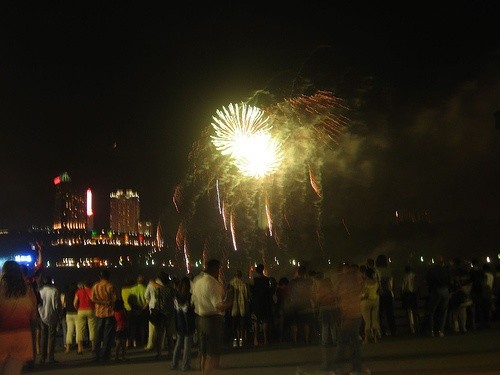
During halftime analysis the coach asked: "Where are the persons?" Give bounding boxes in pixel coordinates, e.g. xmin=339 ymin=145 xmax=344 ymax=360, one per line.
xmin=19 ymin=242 xmax=500 ymax=374
xmin=0 ymin=259 xmax=42 ymax=374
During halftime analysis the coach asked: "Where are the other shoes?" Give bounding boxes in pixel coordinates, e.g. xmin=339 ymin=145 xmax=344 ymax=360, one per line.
xmin=252 ymin=340 xmax=258 ymax=346
xmin=233 ymin=340 xmax=238 ymax=347
xmin=167 ymin=353 xmax=172 ymax=360
xmin=239 ymin=339 xmax=245 ymax=347
xmin=38 ymin=360 xmax=46 ymax=367
xmin=76 ymin=349 xmax=84 ymax=356
xmin=169 ymin=364 xmax=179 ymax=371
xmin=263 ymin=339 xmax=268 ymax=346
xmin=181 ymin=364 xmax=192 ymax=371
xmin=47 ymin=360 xmax=56 ymax=367
xmin=155 ymin=354 xmax=161 ymax=360
xmin=64 ymin=350 xmax=72 ymax=354
